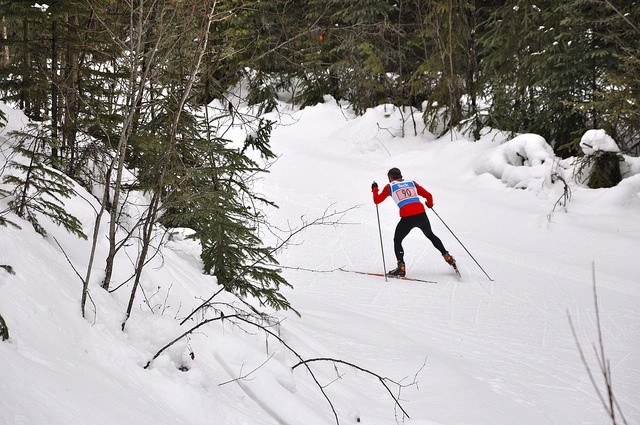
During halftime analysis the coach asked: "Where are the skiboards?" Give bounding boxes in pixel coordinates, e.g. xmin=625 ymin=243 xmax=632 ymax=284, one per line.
xmin=365 ymin=264 xmax=461 ymax=283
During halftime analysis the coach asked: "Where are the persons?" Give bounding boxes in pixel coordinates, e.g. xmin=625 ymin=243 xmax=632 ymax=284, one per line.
xmin=371 ymin=167 xmax=457 ymax=276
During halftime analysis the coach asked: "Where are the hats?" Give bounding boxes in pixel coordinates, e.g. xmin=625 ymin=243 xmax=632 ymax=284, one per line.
xmin=388 ymin=168 xmax=402 ymax=177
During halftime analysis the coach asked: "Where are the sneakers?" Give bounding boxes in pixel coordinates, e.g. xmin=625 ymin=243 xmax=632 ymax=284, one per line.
xmin=389 ymin=262 xmax=405 ymax=277
xmin=443 ymin=252 xmax=456 ymax=266
